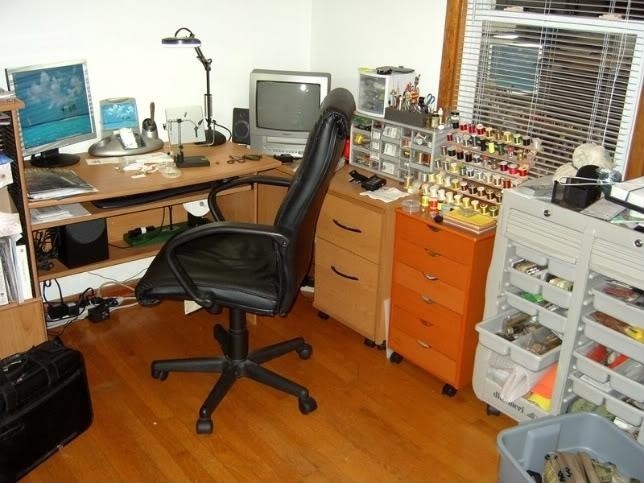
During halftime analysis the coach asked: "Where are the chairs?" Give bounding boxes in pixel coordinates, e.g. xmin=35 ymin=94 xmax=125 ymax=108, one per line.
xmin=136 ymin=87 xmax=352 ymax=432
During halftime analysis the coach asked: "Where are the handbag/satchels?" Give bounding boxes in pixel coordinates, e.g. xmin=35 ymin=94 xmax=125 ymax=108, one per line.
xmin=0 ymin=336 xmax=94 ymax=482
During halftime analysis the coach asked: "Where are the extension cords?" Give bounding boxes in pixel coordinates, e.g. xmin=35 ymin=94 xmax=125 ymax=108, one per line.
xmin=46 ymin=297 xmax=121 ymax=328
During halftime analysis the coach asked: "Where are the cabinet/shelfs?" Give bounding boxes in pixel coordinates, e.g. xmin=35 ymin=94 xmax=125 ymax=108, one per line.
xmin=387 ymin=197 xmax=496 ymax=395
xmin=473 ymin=166 xmax=644 ymax=446
xmin=0 ymin=94 xmax=48 ymax=357
xmin=419 ymin=116 xmax=543 ymax=218
xmin=255 ymin=155 xmax=315 ymax=284
xmin=348 ymin=102 xmax=452 ymax=187
xmin=315 ymin=161 xmax=421 ymax=350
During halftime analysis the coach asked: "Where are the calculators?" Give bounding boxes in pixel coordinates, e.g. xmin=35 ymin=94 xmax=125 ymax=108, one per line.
xmin=362 ymin=174 xmax=387 ymax=191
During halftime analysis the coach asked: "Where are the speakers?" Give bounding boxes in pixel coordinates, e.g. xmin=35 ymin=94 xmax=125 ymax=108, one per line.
xmin=53 ymin=218 xmax=109 ymax=269
xmin=232 ymin=107 xmax=250 ymax=144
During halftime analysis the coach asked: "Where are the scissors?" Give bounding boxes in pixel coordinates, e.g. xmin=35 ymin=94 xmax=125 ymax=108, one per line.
xmin=426 ymin=93 xmax=435 ymax=105
xmin=228 ymin=155 xmax=246 ymax=164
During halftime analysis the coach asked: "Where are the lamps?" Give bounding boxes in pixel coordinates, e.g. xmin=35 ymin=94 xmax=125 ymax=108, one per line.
xmin=159 ymin=27 xmax=234 ymax=146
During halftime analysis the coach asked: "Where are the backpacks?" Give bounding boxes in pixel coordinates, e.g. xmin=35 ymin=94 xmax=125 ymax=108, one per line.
xmin=526 ymin=450 xmax=626 ymax=482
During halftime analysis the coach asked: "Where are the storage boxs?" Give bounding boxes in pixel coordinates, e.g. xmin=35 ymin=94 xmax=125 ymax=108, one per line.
xmin=385 ymin=106 xmax=434 ymax=127
xmin=493 ymin=411 xmax=642 ymax=482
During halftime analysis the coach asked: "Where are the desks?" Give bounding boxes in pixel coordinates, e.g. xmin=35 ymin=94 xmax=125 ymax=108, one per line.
xmin=20 ymin=134 xmax=283 ymax=325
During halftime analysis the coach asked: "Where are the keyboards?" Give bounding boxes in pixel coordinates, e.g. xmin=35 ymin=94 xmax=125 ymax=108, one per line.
xmin=92 ymin=182 xmax=209 ymax=209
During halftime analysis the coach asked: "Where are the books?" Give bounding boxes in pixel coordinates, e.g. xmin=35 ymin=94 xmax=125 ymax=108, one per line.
xmin=23 ymin=168 xmax=97 ymax=198
xmin=0 ymin=238 xmax=29 ymax=306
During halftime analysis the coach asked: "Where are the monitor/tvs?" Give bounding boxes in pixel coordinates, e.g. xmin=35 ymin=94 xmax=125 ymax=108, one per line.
xmin=6 ymin=58 xmax=97 ymax=168
xmin=248 ymin=68 xmax=331 ymax=158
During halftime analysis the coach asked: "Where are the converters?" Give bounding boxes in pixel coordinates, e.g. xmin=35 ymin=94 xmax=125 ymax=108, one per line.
xmin=88 ymin=305 xmax=109 ymax=323
xmin=65 ymin=301 xmax=79 ymax=315
xmin=50 ymin=302 xmax=65 ymax=318
